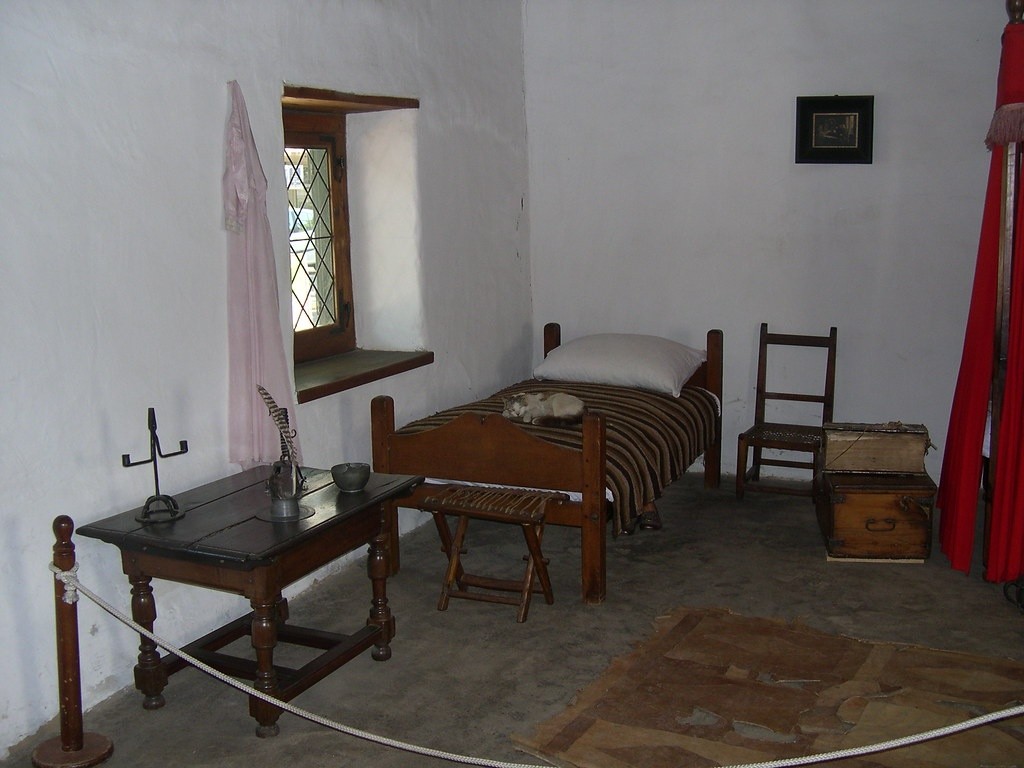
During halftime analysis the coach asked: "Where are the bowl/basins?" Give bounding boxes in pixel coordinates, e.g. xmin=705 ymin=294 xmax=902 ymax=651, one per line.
xmin=331 ymin=463 xmax=371 ymax=492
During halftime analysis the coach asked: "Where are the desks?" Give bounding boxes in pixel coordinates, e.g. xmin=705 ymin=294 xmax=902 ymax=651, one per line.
xmin=74 ymin=463 xmax=425 ymax=737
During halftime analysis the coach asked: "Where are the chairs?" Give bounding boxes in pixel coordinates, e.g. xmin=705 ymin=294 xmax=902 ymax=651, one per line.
xmin=733 ymin=322 xmax=838 ymax=501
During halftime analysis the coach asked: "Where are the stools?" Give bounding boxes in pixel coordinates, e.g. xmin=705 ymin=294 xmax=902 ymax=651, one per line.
xmin=421 ymin=484 xmax=570 ymax=622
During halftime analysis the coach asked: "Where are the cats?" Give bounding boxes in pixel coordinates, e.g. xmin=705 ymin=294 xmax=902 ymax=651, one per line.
xmin=500 ymin=390 xmax=587 ymax=428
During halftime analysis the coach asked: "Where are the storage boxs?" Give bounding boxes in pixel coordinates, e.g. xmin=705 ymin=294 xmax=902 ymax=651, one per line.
xmin=815 ymin=422 xmax=938 ymax=561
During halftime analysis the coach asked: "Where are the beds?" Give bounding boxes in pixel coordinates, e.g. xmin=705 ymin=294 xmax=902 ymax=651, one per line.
xmin=369 ymin=321 xmax=724 ymax=602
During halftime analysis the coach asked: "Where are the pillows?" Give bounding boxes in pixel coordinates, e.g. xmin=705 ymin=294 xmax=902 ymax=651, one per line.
xmin=533 ymin=333 xmax=708 ymax=398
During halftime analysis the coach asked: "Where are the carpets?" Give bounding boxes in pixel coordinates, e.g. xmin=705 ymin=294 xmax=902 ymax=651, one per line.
xmin=510 ymin=605 xmax=1024 ymax=768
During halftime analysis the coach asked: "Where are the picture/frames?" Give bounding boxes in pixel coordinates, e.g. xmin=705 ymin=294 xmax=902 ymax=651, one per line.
xmin=795 ymin=94 xmax=874 ymax=164
